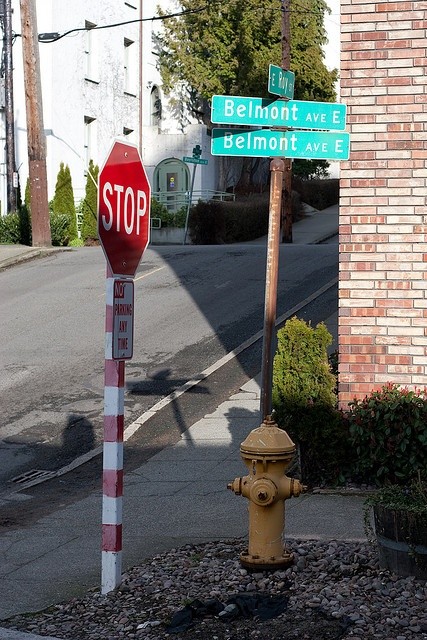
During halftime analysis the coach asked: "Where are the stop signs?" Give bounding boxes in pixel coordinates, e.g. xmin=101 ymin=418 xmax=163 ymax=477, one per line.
xmin=97 ymin=141 xmax=152 ymax=279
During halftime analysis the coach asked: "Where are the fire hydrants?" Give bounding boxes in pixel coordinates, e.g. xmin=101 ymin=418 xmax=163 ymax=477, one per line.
xmin=228 ymin=410 xmax=311 ymax=569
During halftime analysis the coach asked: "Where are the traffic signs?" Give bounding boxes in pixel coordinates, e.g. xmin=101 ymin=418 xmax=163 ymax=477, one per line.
xmin=201 ymin=126 xmax=350 ymax=162
xmin=208 ymin=92 xmax=349 ymax=129
xmin=269 ymin=63 xmax=297 ymax=99
xmin=183 ymin=156 xmax=207 ymax=165
